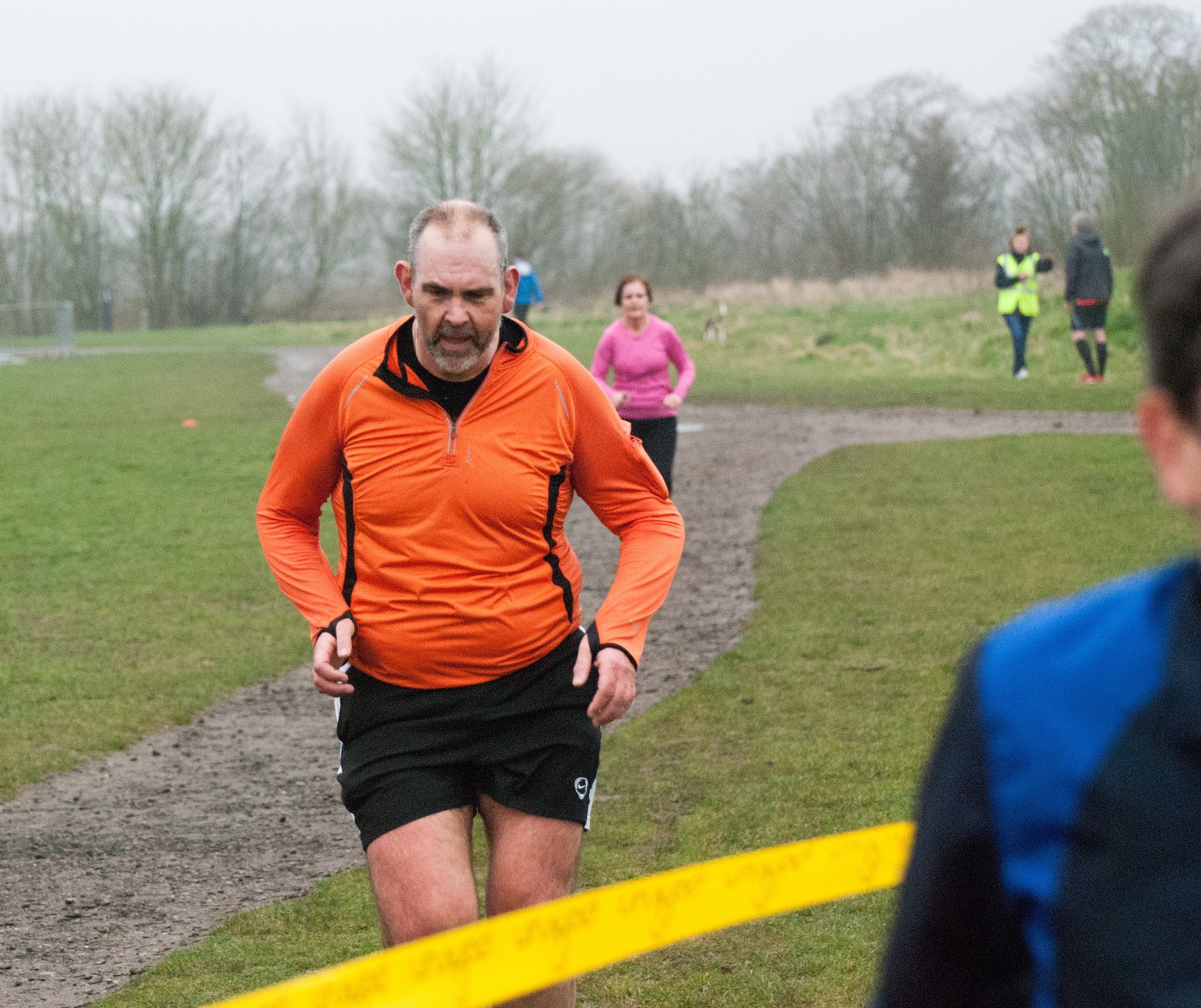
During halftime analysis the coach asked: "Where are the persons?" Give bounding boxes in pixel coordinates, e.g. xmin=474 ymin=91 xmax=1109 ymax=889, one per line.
xmin=878 ymin=203 xmax=1201 ymax=1008
xmin=994 ymin=226 xmax=1054 ymax=380
xmin=1063 ymin=211 xmax=1114 ymax=380
xmin=253 ymin=200 xmax=695 ymax=1008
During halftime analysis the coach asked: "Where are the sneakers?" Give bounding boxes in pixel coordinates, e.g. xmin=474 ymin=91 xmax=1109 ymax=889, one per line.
xmin=1014 ymin=368 xmax=1028 ymax=379
xmin=1080 ymin=372 xmax=1096 ymax=384
xmin=1093 ymin=374 xmax=1104 ymax=381
